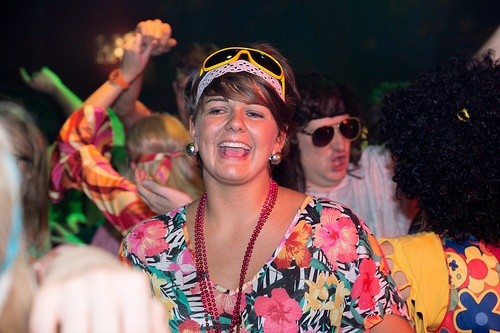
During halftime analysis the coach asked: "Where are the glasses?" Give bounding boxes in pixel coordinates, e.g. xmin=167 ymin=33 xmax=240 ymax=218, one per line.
xmin=200 ymin=46 xmax=285 ymax=102
xmin=300 ymin=117 xmax=362 ymax=148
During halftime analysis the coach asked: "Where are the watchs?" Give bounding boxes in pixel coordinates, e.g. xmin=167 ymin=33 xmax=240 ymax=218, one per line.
xmin=109 ymin=69 xmax=128 ymax=89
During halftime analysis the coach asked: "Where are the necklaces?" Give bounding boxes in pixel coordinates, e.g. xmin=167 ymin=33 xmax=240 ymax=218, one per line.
xmin=193 ymin=180 xmax=280 ymax=333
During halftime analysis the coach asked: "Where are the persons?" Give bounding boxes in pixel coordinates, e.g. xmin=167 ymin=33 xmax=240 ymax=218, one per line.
xmin=0 ymin=101 xmax=173 ymax=333
xmin=19 ymin=18 xmax=413 ymax=258
xmin=112 ymin=43 xmax=412 ymax=333
xmin=374 ymin=27 xmax=500 ymax=333
